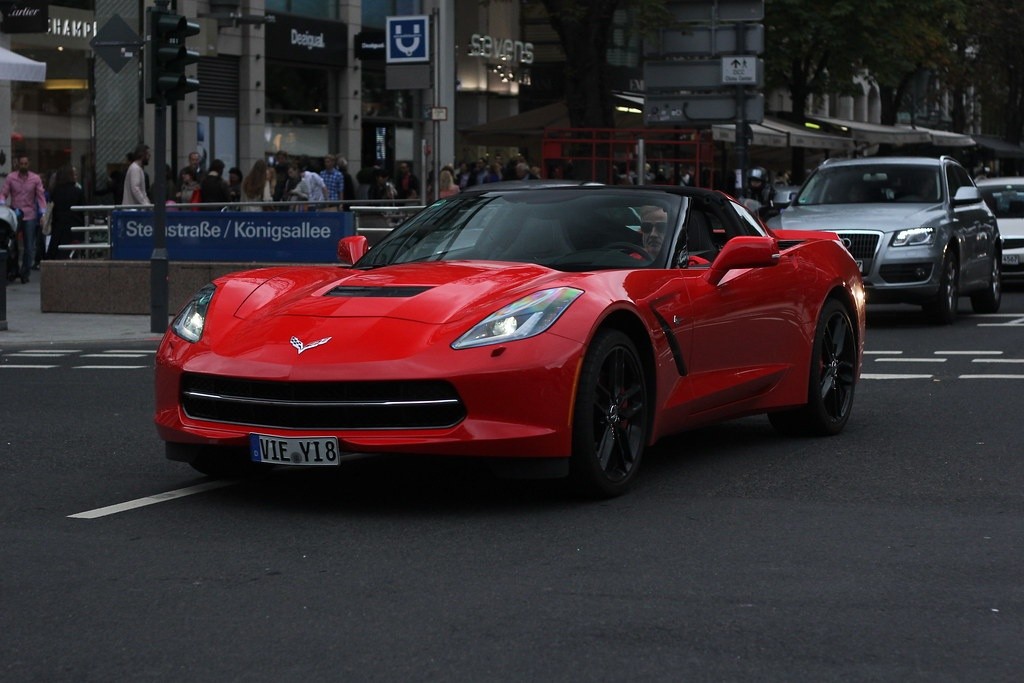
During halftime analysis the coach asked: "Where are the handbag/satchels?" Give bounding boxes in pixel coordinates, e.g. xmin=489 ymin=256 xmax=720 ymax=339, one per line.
xmin=191 ymin=187 xmax=203 ymax=211
xmin=41 ymin=202 xmax=54 ymax=235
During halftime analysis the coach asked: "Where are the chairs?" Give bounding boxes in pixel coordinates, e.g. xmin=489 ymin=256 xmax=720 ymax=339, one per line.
xmin=482 ymin=215 xmax=721 ymax=272
xmin=983 ymin=195 xmax=1024 ymax=219
xmin=837 ymin=174 xmax=957 ymax=205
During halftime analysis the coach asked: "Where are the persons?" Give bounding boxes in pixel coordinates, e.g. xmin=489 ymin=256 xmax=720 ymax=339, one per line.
xmin=48 ymin=145 xmax=460 ymax=260
xmin=629 ymin=204 xmax=712 ymax=266
xmin=0 ymin=154 xmax=47 ymax=285
xmin=897 ymin=176 xmax=939 ymax=201
xmin=455 ymin=152 xmax=808 ymax=208
xmin=832 ymin=183 xmax=867 ymax=205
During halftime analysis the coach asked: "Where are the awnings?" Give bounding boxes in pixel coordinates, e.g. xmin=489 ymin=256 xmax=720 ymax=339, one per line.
xmin=710 ymin=116 xmax=1024 ymax=159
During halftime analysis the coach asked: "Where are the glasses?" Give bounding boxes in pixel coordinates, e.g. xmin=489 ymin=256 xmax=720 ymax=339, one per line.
xmin=641 ymin=222 xmax=667 ymax=234
xmin=399 ymin=166 xmax=406 ymax=170
xmin=19 ymin=162 xmax=28 ymax=166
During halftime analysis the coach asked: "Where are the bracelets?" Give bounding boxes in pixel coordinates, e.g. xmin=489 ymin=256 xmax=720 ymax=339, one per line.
xmin=41 ymin=214 xmax=46 ymax=217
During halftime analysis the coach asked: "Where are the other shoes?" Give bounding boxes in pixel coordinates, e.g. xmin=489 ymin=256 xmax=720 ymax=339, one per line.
xmin=21 ymin=276 xmax=30 ymax=284
xmin=32 ymin=264 xmax=39 ymax=271
xmin=8 ymin=273 xmax=16 ymax=282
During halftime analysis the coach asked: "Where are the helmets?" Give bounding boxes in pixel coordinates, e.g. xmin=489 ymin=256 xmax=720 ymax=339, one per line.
xmin=746 ymin=166 xmax=768 ymax=183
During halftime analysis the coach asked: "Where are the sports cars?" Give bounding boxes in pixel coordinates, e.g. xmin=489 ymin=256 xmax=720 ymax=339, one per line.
xmin=156 ymin=186 xmax=863 ymax=503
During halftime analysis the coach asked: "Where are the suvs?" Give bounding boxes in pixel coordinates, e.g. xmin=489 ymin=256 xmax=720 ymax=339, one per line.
xmin=975 ymin=174 xmax=1023 ymax=290
xmin=764 ymin=156 xmax=1002 ymax=317
